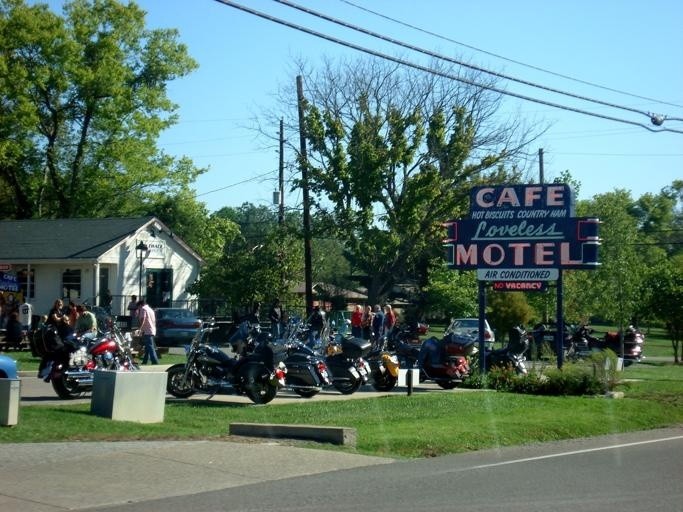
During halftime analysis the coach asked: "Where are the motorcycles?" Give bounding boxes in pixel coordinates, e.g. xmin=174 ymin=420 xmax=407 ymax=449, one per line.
xmin=0 ymin=356 xmax=17 ymax=378
xmin=567 ymin=323 xmax=645 ymax=367
xmin=32 ymin=315 xmax=139 ymax=399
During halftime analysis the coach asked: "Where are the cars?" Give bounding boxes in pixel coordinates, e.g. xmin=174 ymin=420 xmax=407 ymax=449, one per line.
xmin=152 ymin=307 xmax=202 ymax=346
xmin=528 ymin=321 xmax=575 ymax=360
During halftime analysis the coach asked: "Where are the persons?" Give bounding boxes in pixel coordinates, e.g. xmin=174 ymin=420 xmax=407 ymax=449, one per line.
xmin=249 ymin=297 xmax=396 ymax=352
xmin=6 ymin=288 xmax=159 ymax=364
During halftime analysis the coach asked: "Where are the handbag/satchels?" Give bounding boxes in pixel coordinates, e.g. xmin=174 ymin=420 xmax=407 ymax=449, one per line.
xmin=28 ymin=326 xmax=64 ymax=357
